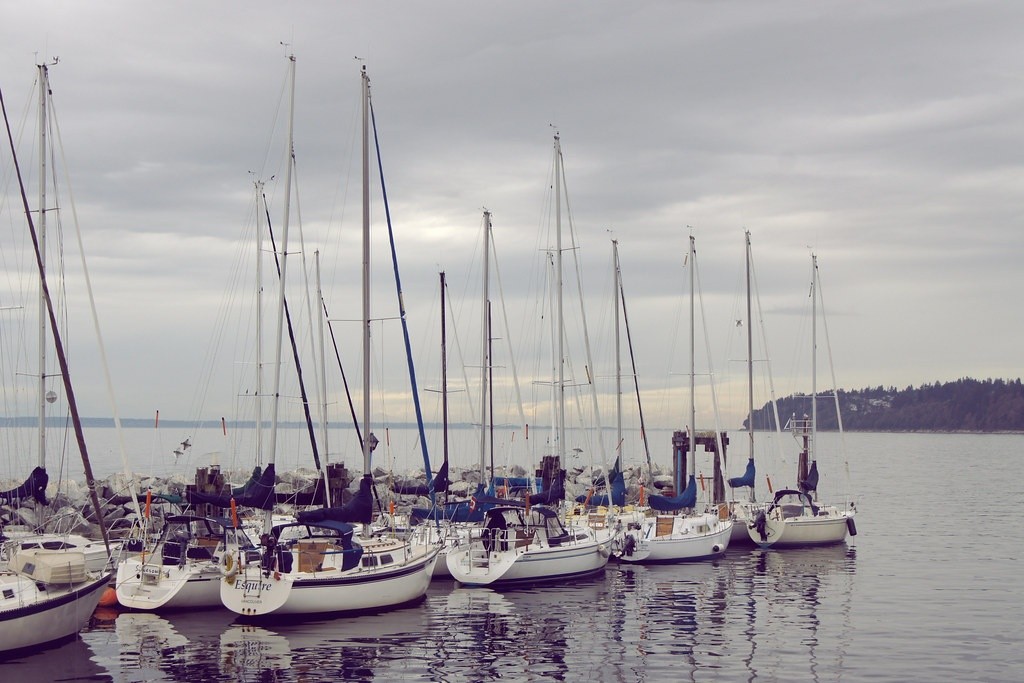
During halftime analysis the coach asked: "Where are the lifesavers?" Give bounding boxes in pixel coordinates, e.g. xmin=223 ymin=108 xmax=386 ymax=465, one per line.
xmin=377 ymin=513 xmax=391 ymax=527
xmin=219 ymin=551 xmax=238 ymax=576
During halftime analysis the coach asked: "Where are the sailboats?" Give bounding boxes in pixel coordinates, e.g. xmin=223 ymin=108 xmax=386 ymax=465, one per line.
xmin=0 ymin=44 xmax=858 ymax=663
